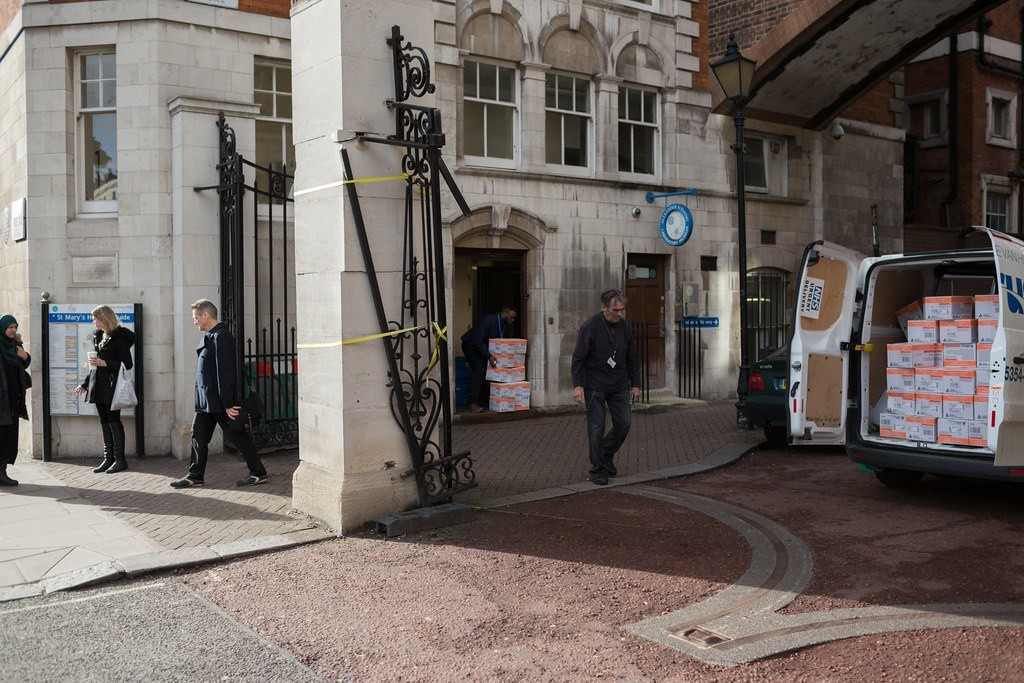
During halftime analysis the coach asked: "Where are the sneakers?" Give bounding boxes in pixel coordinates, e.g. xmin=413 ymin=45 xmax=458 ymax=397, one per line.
xmin=238 ymin=474 xmax=269 ymax=486
xmin=171 ymin=474 xmax=204 ymax=488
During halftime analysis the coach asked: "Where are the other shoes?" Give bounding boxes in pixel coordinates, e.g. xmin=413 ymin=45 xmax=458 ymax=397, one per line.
xmin=592 ymin=475 xmax=609 ymax=485
xmin=604 ymin=454 xmax=617 ymax=477
xmin=465 ymin=404 xmax=484 ymax=413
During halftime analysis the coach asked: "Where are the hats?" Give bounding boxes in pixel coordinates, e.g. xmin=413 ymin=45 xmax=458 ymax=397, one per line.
xmin=0 ymin=313 xmax=19 ymax=337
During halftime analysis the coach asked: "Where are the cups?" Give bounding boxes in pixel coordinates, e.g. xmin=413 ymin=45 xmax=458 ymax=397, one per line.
xmin=88 ymin=351 xmax=97 ymax=370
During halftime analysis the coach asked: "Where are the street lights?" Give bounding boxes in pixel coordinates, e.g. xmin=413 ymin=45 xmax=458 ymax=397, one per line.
xmin=707 ymin=28 xmax=760 ymax=430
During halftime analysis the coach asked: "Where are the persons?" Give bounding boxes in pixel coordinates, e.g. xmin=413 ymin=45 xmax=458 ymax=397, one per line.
xmin=571 ymin=290 xmax=641 ymax=485
xmin=75 ymin=304 xmax=137 ymax=473
xmin=0 ymin=314 xmax=32 ymax=486
xmin=170 ymin=298 xmax=270 ymax=489
xmin=460 ymin=307 xmax=516 ymax=412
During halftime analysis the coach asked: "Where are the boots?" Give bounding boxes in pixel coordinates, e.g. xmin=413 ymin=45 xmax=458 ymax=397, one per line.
xmin=105 ymin=422 xmax=128 ymax=473
xmin=93 ymin=423 xmax=115 ymax=473
xmin=0 ymin=463 xmax=19 ymax=486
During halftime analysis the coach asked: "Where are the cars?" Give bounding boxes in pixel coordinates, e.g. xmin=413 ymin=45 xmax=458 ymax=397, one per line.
xmin=745 ymin=339 xmax=788 ymax=446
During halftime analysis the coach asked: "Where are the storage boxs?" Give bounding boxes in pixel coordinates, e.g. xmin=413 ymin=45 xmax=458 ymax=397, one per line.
xmin=490 ymin=396 xmax=529 ymax=411
xmin=490 ymin=382 xmax=531 ymax=397
xmin=489 ymin=338 xmax=527 ymax=354
xmin=455 ymin=356 xmax=472 ymax=406
xmin=486 ymin=367 xmax=526 ymax=382
xmin=879 ymin=293 xmax=1000 ymax=446
xmin=487 ymin=354 xmax=526 ymax=368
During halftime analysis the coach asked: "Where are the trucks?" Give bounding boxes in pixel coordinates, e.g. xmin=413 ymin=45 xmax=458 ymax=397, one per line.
xmin=781 ymin=225 xmax=1024 ymax=490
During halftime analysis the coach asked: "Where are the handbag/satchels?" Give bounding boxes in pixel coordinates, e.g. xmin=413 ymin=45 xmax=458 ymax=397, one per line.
xmin=233 ymin=392 xmax=263 ymax=431
xmin=110 ymin=363 xmax=138 ymax=410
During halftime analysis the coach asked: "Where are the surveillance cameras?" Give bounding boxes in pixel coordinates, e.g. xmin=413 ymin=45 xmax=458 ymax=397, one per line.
xmin=831 ymin=125 xmax=844 ymax=140
xmin=632 ymin=207 xmax=642 ymax=217
